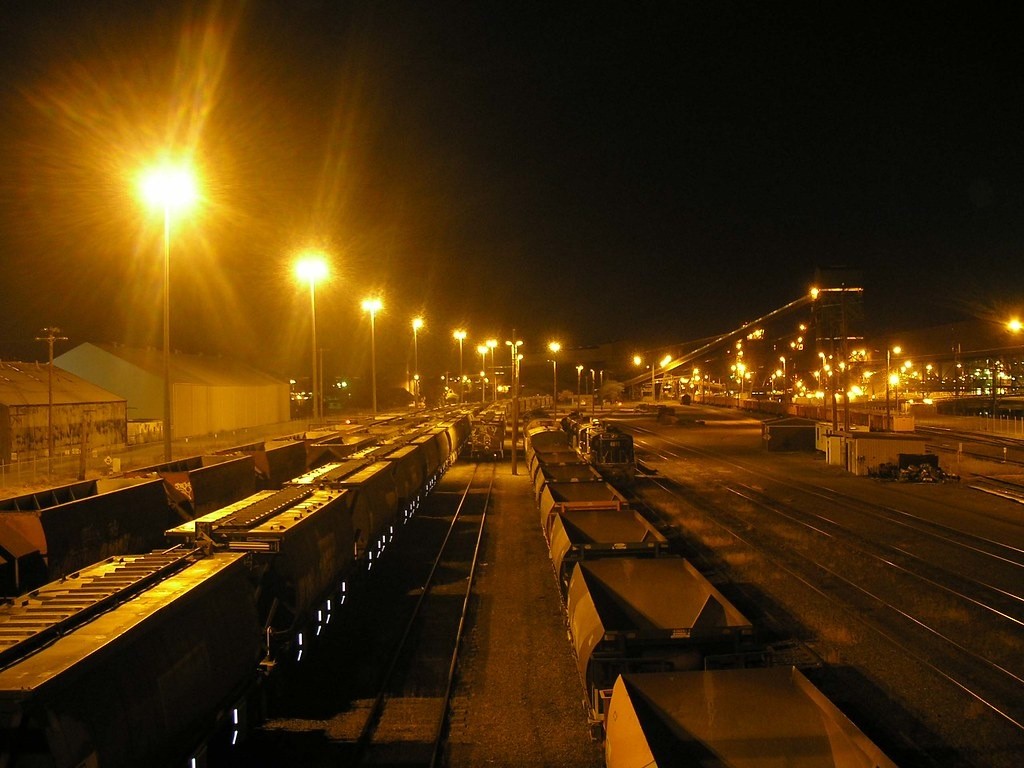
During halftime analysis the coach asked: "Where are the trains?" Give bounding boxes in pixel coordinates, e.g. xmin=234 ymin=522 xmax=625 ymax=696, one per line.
xmin=693 ymin=392 xmax=916 ymax=433
xmin=1 ymin=399 xmax=491 ymax=768
xmin=467 ymin=391 xmax=552 ymax=462
xmin=522 ymin=406 xmax=898 ymax=768
xmin=1 ymin=401 xmax=476 ymax=605
xmin=561 ymin=410 xmax=636 ymax=490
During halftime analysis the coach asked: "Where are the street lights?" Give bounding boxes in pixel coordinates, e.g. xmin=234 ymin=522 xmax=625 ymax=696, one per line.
xmin=779 ymin=356 xmax=786 ymax=393
xmin=412 ymin=316 xmax=426 ymax=408
xmin=599 ymin=369 xmax=605 ymax=410
xmin=34 ymin=325 xmax=69 ymax=486
xmin=544 ymin=359 xmax=558 ymax=422
xmin=886 ymin=346 xmax=901 ymax=433
xmin=819 ymin=351 xmax=825 ymax=386
xmin=293 ymin=250 xmax=330 ymax=419
xmin=504 ymin=340 xmax=525 ymax=397
xmin=889 ymin=373 xmax=899 ymax=411
xmin=359 ymin=297 xmax=385 ymax=414
xmin=548 ymin=342 xmax=560 ymax=395
xmin=135 ymin=158 xmax=201 ymax=463
xmin=589 ymin=368 xmax=595 ymax=415
xmin=771 ymin=373 xmax=776 ymax=400
xmin=486 ymin=338 xmax=498 ymax=400
xmin=575 ymin=365 xmax=583 ymax=408
xmin=817 ymin=364 xmax=830 ymax=388
xmin=477 ymin=345 xmax=488 ymax=403
xmin=453 ymin=329 xmax=468 ymax=403
